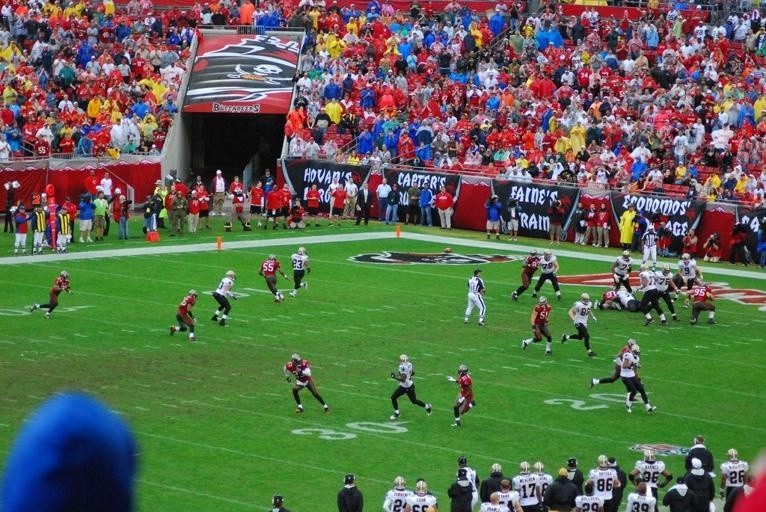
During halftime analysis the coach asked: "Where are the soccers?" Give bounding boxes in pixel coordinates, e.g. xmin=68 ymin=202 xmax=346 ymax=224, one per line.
xmin=445 ymin=248 xmax=451 ymax=252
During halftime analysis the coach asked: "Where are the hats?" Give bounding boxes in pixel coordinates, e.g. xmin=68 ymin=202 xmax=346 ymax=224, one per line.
xmin=0 ymin=0 xmax=766 ymax=247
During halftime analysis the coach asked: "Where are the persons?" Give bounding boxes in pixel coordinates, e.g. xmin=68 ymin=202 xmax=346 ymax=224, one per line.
xmin=446 ymin=365 xmax=475 ymax=427
xmin=211 ymin=270 xmax=238 ymax=328
xmin=270 ymin=436 xmax=766 ymax=511
xmin=1 ymin=2 xmax=766 ymax=270
xmin=30 ymin=271 xmax=72 ymax=319
xmin=1 ymin=391 xmax=140 ymax=511
xmin=511 ymin=250 xmax=718 ymax=356
xmin=258 ymin=247 xmax=311 ymax=303
xmin=464 ymin=269 xmax=488 ymax=326
xmin=283 ymin=354 xmax=329 ymax=413
xmin=589 ymin=339 xmax=657 ymax=415
xmin=170 ymin=289 xmax=198 ymax=341
xmin=390 ymin=354 xmax=433 ymax=420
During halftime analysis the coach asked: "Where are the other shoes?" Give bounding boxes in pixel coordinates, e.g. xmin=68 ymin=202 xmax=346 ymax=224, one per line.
xmin=29 ymin=248 xmax=720 ymax=430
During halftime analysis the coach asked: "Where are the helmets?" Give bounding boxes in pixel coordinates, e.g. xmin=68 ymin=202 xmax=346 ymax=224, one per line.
xmin=269 ymin=434 xmax=740 ymax=512
